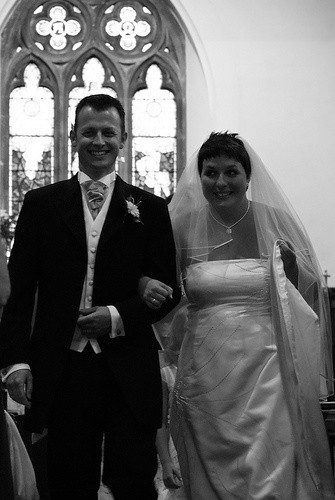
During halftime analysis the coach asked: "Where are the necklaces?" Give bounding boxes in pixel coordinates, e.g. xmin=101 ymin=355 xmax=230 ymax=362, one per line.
xmin=210 ymin=200 xmax=250 ymax=235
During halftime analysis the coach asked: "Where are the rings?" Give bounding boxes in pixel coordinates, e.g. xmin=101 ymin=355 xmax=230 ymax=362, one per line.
xmin=152 ymin=299 xmax=155 ymax=304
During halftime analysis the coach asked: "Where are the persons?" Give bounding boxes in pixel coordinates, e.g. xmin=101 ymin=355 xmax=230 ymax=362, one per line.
xmin=137 ymin=130 xmax=334 ymax=500
xmin=0 ymin=237 xmax=39 ymax=500
xmin=0 ymin=94 xmax=182 ymax=500
xmin=153 ymin=303 xmax=192 ymax=500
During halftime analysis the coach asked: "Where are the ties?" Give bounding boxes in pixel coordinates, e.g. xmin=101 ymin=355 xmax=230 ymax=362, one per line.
xmin=81 ymin=180 xmax=108 ymax=220
